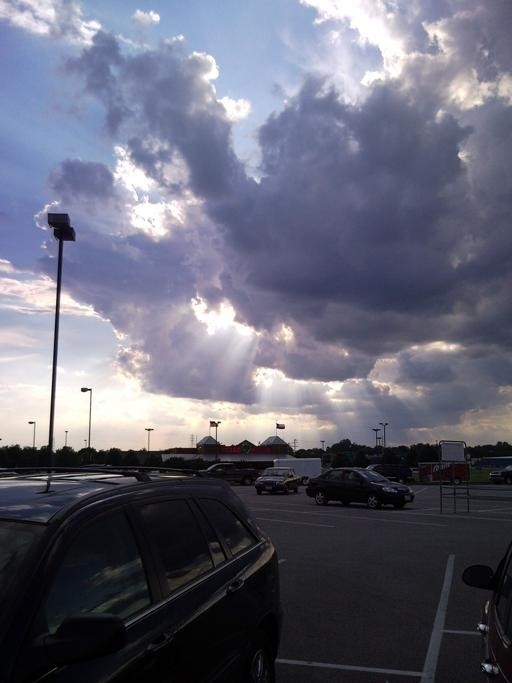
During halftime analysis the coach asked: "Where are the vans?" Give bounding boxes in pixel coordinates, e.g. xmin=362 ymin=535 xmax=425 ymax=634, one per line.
xmin=365 ymin=462 xmax=414 ymax=482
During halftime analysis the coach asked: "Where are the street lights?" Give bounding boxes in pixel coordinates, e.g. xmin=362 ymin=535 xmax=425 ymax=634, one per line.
xmin=80 ymin=387 xmax=92 ymax=447
xmin=65 ymin=429 xmax=69 ymax=444
xmin=378 ymin=421 xmax=389 ymax=446
xmin=377 ymin=437 xmax=382 ymax=446
xmin=371 ymin=427 xmax=382 ymax=446
xmin=144 ymin=427 xmax=154 ymax=450
xmin=28 ymin=420 xmax=37 ymax=447
xmin=46 ymin=211 xmax=80 ymax=465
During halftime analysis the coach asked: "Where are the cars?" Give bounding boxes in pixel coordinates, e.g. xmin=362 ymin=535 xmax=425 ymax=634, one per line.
xmin=303 ymin=464 xmax=417 ymax=510
xmin=462 ymin=537 xmax=512 ymax=682
xmin=253 ymin=465 xmax=301 ymax=495
xmin=487 ymin=464 xmax=512 ymax=483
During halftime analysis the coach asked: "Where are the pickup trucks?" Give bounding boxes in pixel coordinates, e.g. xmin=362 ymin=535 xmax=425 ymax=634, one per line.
xmin=192 ymin=461 xmax=257 ymax=485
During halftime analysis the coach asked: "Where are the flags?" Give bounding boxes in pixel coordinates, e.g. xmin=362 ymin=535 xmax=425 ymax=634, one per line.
xmin=276 ymin=423 xmax=285 ymax=429
xmin=210 ymin=421 xmax=218 ymax=427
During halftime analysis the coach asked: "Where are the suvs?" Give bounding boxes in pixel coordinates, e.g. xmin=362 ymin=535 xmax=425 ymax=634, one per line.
xmin=0 ymin=464 xmax=288 ymax=682
